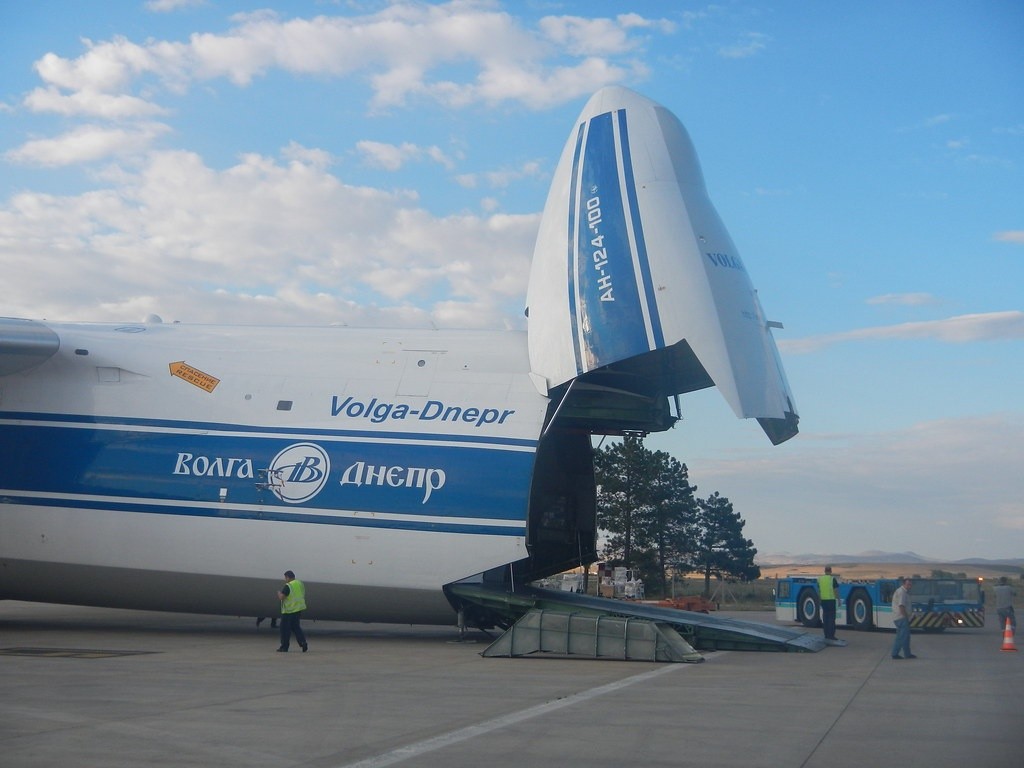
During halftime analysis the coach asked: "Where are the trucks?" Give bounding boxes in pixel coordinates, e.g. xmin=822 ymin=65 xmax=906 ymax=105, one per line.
xmin=775 ymin=572 xmax=986 ymax=631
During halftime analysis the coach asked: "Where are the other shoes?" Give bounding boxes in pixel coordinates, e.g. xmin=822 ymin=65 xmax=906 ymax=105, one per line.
xmin=271 ymin=624 xmax=279 ymax=628
xmin=906 ymin=654 xmax=916 ymax=658
xmin=302 ymin=642 xmax=307 ymax=652
xmin=892 ymin=655 xmax=904 ymax=659
xmin=825 ymin=636 xmax=838 ymax=640
xmin=277 ymin=646 xmax=287 ymax=652
xmin=256 ymin=617 xmax=260 ymax=627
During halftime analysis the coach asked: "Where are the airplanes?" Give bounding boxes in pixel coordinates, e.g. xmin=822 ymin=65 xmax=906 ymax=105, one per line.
xmin=1 ymin=81 xmax=799 ymax=642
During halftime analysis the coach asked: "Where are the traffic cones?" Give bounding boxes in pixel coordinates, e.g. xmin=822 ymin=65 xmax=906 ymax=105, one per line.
xmin=1000 ymin=616 xmax=1018 ymax=651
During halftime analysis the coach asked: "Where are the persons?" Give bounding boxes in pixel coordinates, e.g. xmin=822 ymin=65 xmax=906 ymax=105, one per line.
xmin=993 ymin=577 xmax=1017 ymax=636
xmin=892 ymin=579 xmax=916 ymax=659
xmin=276 ymin=571 xmax=308 ymax=652
xmin=819 ymin=567 xmax=841 ymax=640
